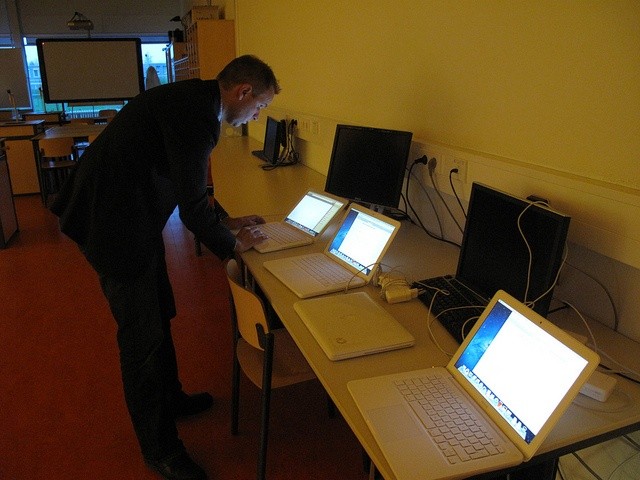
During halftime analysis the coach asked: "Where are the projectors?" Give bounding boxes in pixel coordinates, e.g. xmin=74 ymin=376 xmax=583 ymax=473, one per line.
xmin=65 ymin=20 xmax=94 ymax=30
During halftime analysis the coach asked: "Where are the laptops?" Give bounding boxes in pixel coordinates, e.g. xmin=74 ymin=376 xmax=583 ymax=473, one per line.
xmin=241 ymin=187 xmax=350 ymax=254
xmin=262 ymin=202 xmax=401 ymax=300
xmin=292 ymin=290 xmax=417 ymax=362
xmin=345 ymin=289 xmax=601 ymax=479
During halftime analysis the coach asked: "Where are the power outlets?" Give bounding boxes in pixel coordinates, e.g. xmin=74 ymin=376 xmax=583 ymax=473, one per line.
xmin=412 ymin=148 xmax=442 ymax=177
xmin=301 ymin=119 xmax=319 ymax=135
xmin=288 ymin=117 xmax=299 ymax=131
xmin=448 ymin=157 xmax=468 ymax=183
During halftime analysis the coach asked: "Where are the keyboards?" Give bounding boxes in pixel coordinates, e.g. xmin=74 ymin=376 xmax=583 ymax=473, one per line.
xmin=408 ymin=274 xmax=486 ymax=346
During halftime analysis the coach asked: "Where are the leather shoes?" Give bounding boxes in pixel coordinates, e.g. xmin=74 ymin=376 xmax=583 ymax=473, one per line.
xmin=150 ymin=439 xmax=207 ymax=480
xmin=180 ymin=393 xmax=212 ymax=416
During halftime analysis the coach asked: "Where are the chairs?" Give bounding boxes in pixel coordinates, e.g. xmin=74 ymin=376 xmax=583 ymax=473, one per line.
xmin=98 ymin=110 xmax=116 ymax=122
xmin=34 ymin=136 xmax=80 ymax=210
xmin=71 ymin=117 xmax=91 ymax=127
xmin=224 ymin=273 xmax=317 ymax=479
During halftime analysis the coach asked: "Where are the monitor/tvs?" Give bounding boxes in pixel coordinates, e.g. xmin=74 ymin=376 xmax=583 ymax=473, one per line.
xmin=454 ymin=181 xmax=572 ymax=320
xmin=262 ymin=115 xmax=282 ymax=168
xmin=323 ymin=124 xmax=413 ymax=210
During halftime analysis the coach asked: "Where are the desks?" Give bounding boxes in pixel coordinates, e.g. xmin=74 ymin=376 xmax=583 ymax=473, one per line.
xmin=152 ymin=133 xmax=640 ymax=479
xmin=44 ymin=125 xmax=106 ymax=136
xmin=23 ymin=110 xmax=64 ymax=127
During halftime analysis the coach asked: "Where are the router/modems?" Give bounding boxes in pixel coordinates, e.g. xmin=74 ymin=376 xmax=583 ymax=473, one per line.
xmin=379 ymin=271 xmax=412 ymax=304
xmin=578 ymin=368 xmax=618 ymax=404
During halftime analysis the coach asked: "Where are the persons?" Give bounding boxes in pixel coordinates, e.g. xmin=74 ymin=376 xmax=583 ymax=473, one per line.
xmin=48 ymin=52 xmax=281 ymax=479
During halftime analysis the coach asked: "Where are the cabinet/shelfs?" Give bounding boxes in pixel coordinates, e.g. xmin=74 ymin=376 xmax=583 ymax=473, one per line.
xmin=6 ymin=138 xmax=41 ymax=197
xmin=185 ymin=22 xmax=234 ymax=80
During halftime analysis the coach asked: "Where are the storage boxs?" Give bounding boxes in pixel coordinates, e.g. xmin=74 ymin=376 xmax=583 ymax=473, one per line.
xmin=187 ymin=5 xmax=219 ymax=23
